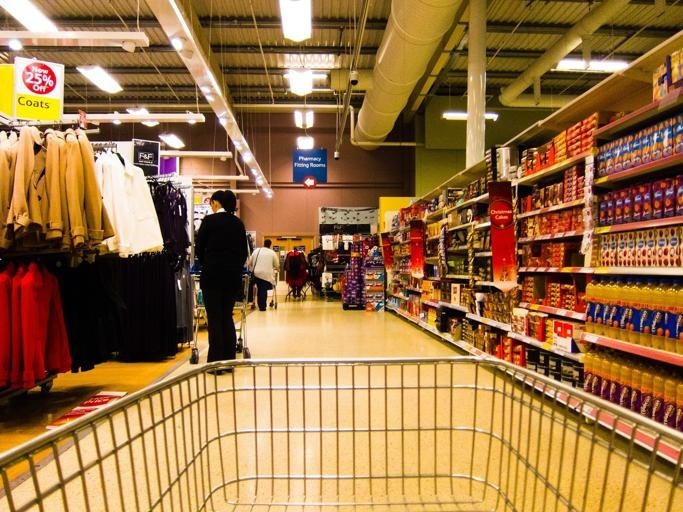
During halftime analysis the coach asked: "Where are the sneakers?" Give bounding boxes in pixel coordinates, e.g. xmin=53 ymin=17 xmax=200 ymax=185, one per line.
xmin=208 ymin=369 xmax=233 ymax=375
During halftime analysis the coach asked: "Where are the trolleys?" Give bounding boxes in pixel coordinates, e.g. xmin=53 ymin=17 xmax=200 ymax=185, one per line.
xmin=187 ymin=261 xmax=252 ymax=364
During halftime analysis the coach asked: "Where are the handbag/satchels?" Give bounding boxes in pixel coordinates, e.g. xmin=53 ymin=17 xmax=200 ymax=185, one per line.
xmin=251 ymin=272 xmax=255 ymax=284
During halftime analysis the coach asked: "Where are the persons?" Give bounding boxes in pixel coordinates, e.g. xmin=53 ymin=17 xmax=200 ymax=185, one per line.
xmin=195 ymin=190 xmax=248 ymax=375
xmin=247 ymin=239 xmax=280 ymax=311
xmin=284 ymin=248 xmax=308 ymax=301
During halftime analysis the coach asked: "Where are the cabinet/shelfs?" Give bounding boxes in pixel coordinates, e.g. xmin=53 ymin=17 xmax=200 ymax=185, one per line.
xmin=387 ymin=36 xmax=682 ymax=467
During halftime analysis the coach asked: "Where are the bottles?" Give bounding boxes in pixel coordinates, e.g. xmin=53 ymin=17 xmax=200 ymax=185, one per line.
xmin=582 ymin=277 xmax=681 ymax=353
xmin=582 ymin=348 xmax=682 ymax=431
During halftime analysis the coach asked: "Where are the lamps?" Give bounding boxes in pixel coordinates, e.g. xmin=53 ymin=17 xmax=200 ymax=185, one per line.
xmin=333 ymin=150 xmax=341 ymax=161
xmin=348 ymin=69 xmax=361 ymax=86
